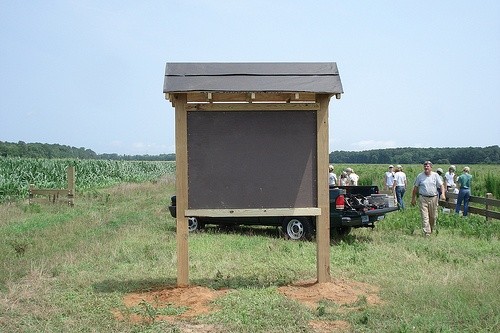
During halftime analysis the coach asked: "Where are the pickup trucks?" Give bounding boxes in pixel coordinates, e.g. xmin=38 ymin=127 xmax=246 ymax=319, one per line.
xmin=169 ymin=186 xmax=400 ymax=240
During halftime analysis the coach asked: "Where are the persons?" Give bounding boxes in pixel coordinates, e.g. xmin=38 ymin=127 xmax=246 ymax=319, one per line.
xmin=436 ymin=165 xmax=473 ymax=218
xmin=384 ymin=165 xmax=407 ymax=210
xmin=329 ymin=165 xmax=359 ymax=186
xmin=411 ymin=161 xmax=446 ymax=237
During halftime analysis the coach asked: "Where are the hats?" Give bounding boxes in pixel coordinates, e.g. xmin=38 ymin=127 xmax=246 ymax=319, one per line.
xmin=450 ymin=165 xmax=456 ymax=171
xmin=342 ymin=171 xmax=347 ymax=175
xmin=329 ymin=165 xmax=334 ymax=170
xmin=464 ymin=167 xmax=469 ymax=171
xmin=437 ymin=168 xmax=443 ymax=172
xmin=397 ymin=165 xmax=402 ymax=168
xmin=346 ymin=168 xmax=352 ymax=171
xmin=389 ymin=165 xmax=393 ymax=167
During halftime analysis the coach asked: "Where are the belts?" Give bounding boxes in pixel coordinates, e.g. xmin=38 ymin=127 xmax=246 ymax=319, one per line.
xmin=419 ymin=194 xmax=437 ymax=197
